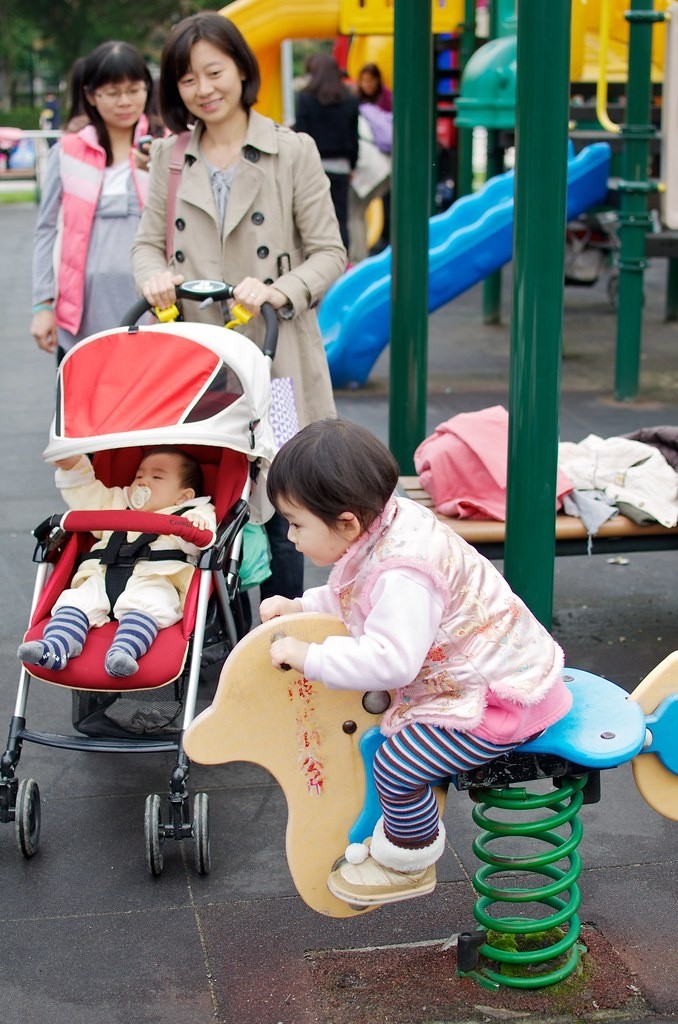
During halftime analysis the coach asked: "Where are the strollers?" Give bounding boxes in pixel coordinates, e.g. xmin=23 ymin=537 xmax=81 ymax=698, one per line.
xmin=4 ymin=280 xmax=280 ymax=879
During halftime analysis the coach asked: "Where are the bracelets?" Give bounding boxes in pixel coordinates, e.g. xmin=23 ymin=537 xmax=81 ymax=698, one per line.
xmin=32 ymin=305 xmax=54 ymax=312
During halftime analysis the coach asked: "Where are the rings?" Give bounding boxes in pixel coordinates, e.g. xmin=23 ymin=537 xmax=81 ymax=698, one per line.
xmin=249 ymin=293 xmax=256 ymax=299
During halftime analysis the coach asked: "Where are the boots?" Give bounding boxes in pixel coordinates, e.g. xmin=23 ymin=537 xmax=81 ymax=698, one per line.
xmin=327 ymin=815 xmax=446 ymax=906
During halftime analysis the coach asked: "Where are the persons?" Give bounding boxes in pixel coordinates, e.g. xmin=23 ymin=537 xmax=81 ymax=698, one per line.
xmin=130 ymin=13 xmax=349 ymax=605
xmin=28 ymin=40 xmax=174 ymax=370
xmin=259 ymin=418 xmax=572 ymax=906
xmin=16 ymin=446 xmax=216 ymax=677
xmin=290 ymin=53 xmax=392 ymax=257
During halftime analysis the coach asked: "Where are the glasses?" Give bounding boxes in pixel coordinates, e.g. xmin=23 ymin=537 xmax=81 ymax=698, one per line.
xmin=95 ymin=83 xmax=147 ymax=103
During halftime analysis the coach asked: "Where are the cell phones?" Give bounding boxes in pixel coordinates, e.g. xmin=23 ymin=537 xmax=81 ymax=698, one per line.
xmin=138 ymin=135 xmax=152 ymax=155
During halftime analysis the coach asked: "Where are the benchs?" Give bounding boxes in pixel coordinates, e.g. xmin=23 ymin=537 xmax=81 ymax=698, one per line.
xmin=396 ymin=476 xmax=678 ymax=560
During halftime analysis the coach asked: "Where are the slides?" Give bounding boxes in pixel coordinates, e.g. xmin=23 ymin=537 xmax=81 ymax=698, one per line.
xmin=317 ymin=136 xmax=613 ymax=392
